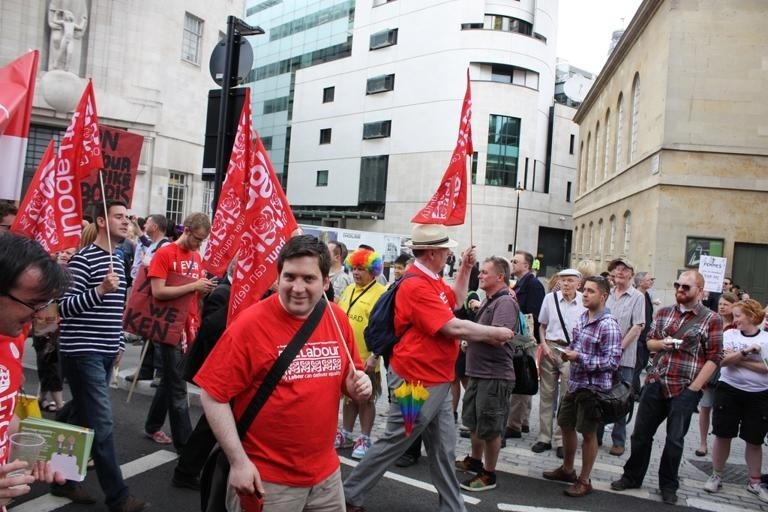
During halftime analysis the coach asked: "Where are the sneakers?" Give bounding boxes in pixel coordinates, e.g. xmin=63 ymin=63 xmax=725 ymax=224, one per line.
xmin=351 ymin=433 xmax=371 ymax=459
xmin=333 ymin=429 xmax=354 ymax=448
xmin=459 ymin=469 xmax=497 ymax=492
xmin=453 ymin=455 xmax=483 ymax=474
xmin=141 ymin=429 xmax=173 ymax=444
xmin=746 ymin=479 xmax=768 ymax=503
xmin=395 ymin=452 xmax=421 ymax=467
xmin=703 ymin=472 xmax=723 ymax=493
xmin=170 ymin=471 xmax=202 ymax=490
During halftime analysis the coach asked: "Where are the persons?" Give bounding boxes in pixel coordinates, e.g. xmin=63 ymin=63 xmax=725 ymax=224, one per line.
xmin=456 ymin=253 xmax=520 ymax=493
xmin=445 ymin=251 xmax=456 ymax=278
xmin=322 ymin=240 xmax=354 ymax=306
xmin=138 ymin=213 xmax=173 ymax=271
xmin=541 ymin=275 xmax=622 ymax=499
xmin=129 ymin=216 xmax=154 ymax=286
xmin=601 ymin=257 xmax=646 ymax=458
xmin=507 ymin=250 xmax=545 ymax=438
xmin=610 ymin=270 xmax=724 ymax=505
xmin=632 ymin=270 xmax=654 ymax=401
xmin=0 ymin=202 xmax=19 ymax=235
xmin=336 ymin=247 xmax=388 ymax=461
xmin=388 ymin=253 xmax=412 ymax=293
xmin=534 ymin=266 xmax=585 ymax=460
xmin=79 ymin=224 xmax=100 ymax=253
xmin=550 ymin=257 xmax=595 ymax=297
xmin=707 ymin=277 xmax=767 ymax=332
xmin=47 ymin=198 xmax=154 ymax=511
xmin=166 ymin=220 xmax=175 ymax=246
xmin=704 ymin=300 xmax=767 ymax=505
xmin=342 ymin=219 xmax=515 ymax=511
xmin=0 ymin=230 xmax=66 ymax=512
xmin=459 ymin=252 xmax=464 ymax=266
xmin=143 ymin=213 xmax=218 ymax=463
xmin=531 ymin=257 xmax=541 ymax=277
xmin=193 ymin=234 xmax=372 ymax=512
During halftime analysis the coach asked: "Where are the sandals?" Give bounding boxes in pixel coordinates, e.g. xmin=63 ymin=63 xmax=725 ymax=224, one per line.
xmin=37 ymin=397 xmax=57 ymax=413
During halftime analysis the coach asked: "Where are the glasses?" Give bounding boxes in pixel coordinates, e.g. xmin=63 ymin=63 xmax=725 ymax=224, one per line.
xmin=673 ymin=282 xmax=695 ymax=291
xmin=4 ymin=292 xmax=57 ymax=314
xmin=510 ymin=258 xmax=526 ymax=264
xmin=189 ymin=229 xmax=209 ymax=242
xmin=723 ymin=281 xmax=733 ymax=285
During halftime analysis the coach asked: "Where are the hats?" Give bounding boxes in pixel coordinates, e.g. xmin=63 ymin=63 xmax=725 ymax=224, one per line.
xmin=607 ymin=257 xmax=635 ymax=271
xmin=557 ymin=268 xmax=582 ymax=279
xmin=400 ymin=222 xmax=458 ymax=248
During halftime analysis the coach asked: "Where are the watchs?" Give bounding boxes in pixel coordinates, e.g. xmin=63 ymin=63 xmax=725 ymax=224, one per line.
xmin=741 ymin=350 xmax=748 ymax=357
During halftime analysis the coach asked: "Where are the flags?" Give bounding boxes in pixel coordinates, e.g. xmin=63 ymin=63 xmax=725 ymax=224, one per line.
xmin=224 ymin=130 xmax=299 ymax=328
xmin=0 ymin=51 xmax=41 ymax=202
xmin=8 ymin=139 xmax=57 ymax=257
xmin=196 ymin=88 xmax=252 ymax=280
xmin=409 ymin=66 xmax=473 ymax=227
xmin=53 ymin=77 xmax=105 ymax=248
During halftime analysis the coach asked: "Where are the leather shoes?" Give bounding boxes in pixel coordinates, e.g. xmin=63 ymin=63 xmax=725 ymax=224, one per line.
xmin=50 ymin=482 xmax=100 ymax=504
xmin=531 ymin=441 xmax=553 ymax=453
xmin=609 ymin=445 xmax=626 ymax=455
xmin=696 ymin=446 xmax=707 ymax=456
xmin=501 ymin=424 xmax=522 ymax=438
xmin=661 ymin=485 xmax=678 ymax=505
xmin=563 ymin=478 xmax=594 ymax=496
xmin=150 ymin=375 xmax=162 ymax=387
xmin=557 ymin=445 xmax=565 ymax=458
xmin=124 ymin=371 xmax=154 ymax=381
xmin=611 ymin=479 xmax=641 ymax=489
xmin=107 ymin=495 xmax=152 ymax=512
xmin=543 ymin=465 xmax=577 ymax=481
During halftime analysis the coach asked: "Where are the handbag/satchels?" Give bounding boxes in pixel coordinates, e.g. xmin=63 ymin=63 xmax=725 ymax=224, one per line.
xmin=121 ymin=247 xmax=197 ymax=347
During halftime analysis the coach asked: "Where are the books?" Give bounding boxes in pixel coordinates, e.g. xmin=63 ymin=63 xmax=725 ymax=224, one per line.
xmin=14 ymin=415 xmax=95 ymax=481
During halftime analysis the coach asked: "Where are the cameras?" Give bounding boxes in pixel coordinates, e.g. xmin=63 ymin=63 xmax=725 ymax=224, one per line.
xmin=668 ymin=337 xmax=684 ymax=348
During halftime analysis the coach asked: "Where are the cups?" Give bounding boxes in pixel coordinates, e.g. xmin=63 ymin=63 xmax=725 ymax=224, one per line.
xmin=6 ymin=430 xmax=45 ymax=477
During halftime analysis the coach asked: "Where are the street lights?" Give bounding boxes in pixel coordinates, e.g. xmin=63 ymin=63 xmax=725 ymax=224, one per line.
xmin=513 ymin=181 xmax=524 ymax=280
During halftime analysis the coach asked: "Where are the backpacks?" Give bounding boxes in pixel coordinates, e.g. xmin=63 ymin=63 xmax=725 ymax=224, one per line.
xmin=362 ymin=272 xmax=426 ymax=359
